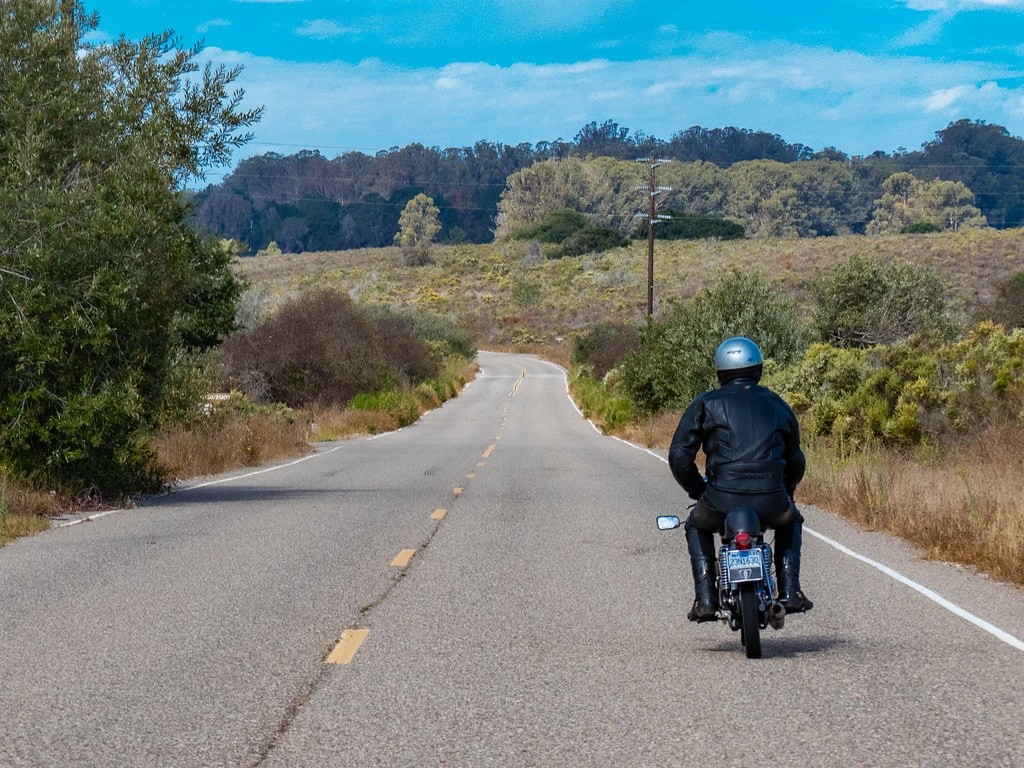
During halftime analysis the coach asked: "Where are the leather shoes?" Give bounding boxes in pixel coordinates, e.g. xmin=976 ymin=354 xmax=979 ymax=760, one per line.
xmin=687 ymin=597 xmax=717 ymax=622
xmin=776 ymin=590 xmax=815 ymax=613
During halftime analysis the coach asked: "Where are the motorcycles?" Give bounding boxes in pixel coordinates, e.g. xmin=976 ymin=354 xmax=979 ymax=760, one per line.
xmin=656 ymin=478 xmax=809 ymax=660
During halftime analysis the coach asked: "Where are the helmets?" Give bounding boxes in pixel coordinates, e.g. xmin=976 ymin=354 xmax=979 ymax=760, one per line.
xmin=711 ymin=336 xmax=765 ymax=373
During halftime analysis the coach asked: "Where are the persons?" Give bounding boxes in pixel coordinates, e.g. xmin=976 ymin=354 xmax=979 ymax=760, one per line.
xmin=668 ymin=337 xmax=815 ymax=620
